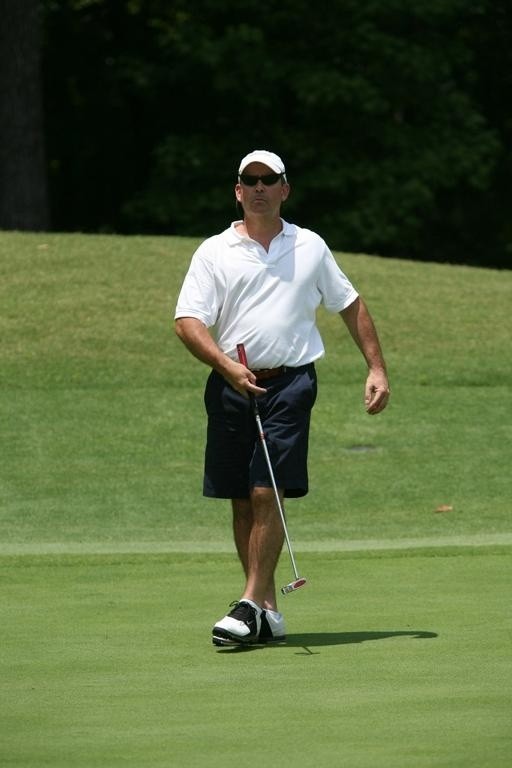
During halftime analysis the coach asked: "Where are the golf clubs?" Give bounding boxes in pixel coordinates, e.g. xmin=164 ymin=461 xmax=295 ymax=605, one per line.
xmin=237 ymin=344 xmax=306 ymax=594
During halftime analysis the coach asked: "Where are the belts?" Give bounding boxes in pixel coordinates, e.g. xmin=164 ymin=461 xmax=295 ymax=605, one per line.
xmin=252 ymin=362 xmax=315 ymax=381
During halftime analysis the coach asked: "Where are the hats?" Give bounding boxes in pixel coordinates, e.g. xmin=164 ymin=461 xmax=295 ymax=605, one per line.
xmin=238 ymin=150 xmax=288 ymax=187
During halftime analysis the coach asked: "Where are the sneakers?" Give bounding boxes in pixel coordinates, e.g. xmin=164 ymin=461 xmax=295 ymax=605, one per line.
xmin=210 ymin=610 xmax=286 ymax=646
xmin=212 ymin=598 xmax=267 ymax=645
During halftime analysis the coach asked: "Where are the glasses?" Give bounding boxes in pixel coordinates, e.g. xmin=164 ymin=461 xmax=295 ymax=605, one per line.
xmin=238 ymin=171 xmax=279 ymax=186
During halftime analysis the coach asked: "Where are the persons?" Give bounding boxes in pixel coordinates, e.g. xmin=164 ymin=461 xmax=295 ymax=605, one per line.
xmin=172 ymin=149 xmax=390 ymax=646
